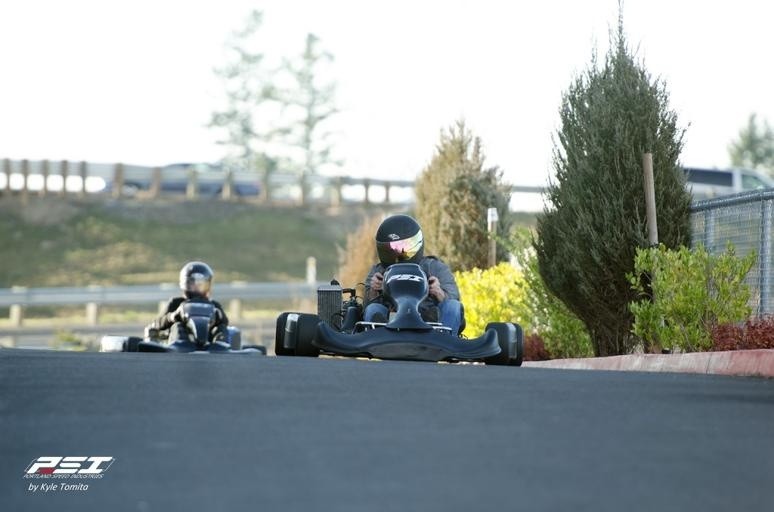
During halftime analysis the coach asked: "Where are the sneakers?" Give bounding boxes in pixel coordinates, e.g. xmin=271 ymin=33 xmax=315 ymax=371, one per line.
xmin=371 ymin=312 xmax=386 ymax=323
xmin=423 ymin=306 xmax=439 ymax=322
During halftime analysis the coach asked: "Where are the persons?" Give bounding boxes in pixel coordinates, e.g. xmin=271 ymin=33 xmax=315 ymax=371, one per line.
xmin=158 ymin=261 xmax=229 ymax=345
xmin=363 ymin=215 xmax=464 ymax=336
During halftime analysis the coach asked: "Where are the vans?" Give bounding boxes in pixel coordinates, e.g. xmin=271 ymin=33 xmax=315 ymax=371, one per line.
xmin=678 ymin=164 xmax=774 ymax=203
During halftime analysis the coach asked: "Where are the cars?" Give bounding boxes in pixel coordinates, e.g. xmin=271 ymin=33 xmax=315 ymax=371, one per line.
xmin=107 ymin=162 xmax=264 ymax=197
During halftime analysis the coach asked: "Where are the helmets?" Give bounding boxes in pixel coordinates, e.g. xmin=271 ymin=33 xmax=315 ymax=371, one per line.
xmin=180 ymin=261 xmax=213 ymax=297
xmin=375 ymin=215 xmax=425 ymax=268
